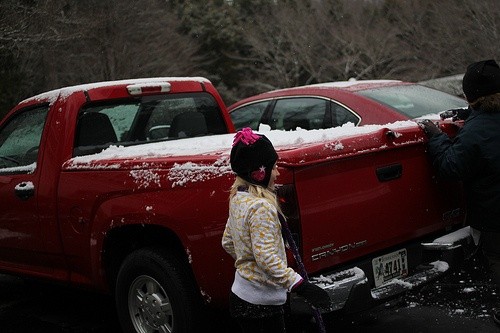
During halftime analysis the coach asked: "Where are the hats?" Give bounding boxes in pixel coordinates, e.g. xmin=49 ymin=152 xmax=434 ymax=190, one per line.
xmin=230 ymin=127 xmax=278 ymax=189
xmin=462 ymin=58 xmax=500 ymax=103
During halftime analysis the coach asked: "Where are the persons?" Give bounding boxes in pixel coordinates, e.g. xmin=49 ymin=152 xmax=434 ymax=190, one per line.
xmin=221 ymin=127 xmax=331 ymax=333
xmin=417 ymin=59 xmax=500 ymax=326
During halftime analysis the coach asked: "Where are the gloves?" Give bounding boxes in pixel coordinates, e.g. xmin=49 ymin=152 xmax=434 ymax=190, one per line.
xmin=440 ymin=108 xmax=470 ymax=122
xmin=418 ymin=118 xmax=443 ymax=139
xmin=293 ymin=280 xmax=331 ymax=309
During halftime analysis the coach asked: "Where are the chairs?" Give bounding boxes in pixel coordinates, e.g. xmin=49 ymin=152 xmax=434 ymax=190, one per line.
xmin=74 ymin=112 xmax=118 ymax=147
xmin=168 ymin=111 xmax=208 ymax=137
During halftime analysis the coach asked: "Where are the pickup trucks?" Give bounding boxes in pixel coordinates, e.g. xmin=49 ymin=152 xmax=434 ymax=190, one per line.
xmin=0 ymin=77 xmax=475 ymax=333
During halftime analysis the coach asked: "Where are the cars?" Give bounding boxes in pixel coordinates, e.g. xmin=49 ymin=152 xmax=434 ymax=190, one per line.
xmin=225 ymin=79 xmax=468 ymax=131
xmin=418 ymin=74 xmax=468 ymax=97
